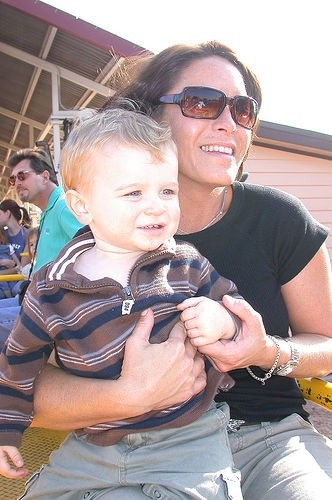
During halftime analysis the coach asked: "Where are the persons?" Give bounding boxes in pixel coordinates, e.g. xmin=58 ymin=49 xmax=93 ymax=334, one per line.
xmin=0 ymin=142 xmax=90 ymax=356
xmin=0 ymin=105 xmax=248 ymax=500
xmin=18 ymin=42 xmax=332 ymax=500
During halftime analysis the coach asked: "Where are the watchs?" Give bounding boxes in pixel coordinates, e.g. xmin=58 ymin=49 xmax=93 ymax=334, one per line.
xmin=262 ymin=335 xmax=301 ymax=378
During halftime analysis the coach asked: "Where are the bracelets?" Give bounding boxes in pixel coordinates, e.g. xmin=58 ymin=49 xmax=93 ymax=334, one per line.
xmin=247 ymin=333 xmax=281 ymax=383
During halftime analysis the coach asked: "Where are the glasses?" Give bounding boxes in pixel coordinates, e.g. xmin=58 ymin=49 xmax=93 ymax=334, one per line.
xmin=153 ymin=86 xmax=259 ymax=129
xmin=7 ymin=170 xmax=36 ymax=186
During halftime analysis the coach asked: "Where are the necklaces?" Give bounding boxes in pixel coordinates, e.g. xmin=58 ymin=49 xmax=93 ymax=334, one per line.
xmin=176 ymin=184 xmax=229 ymax=236
